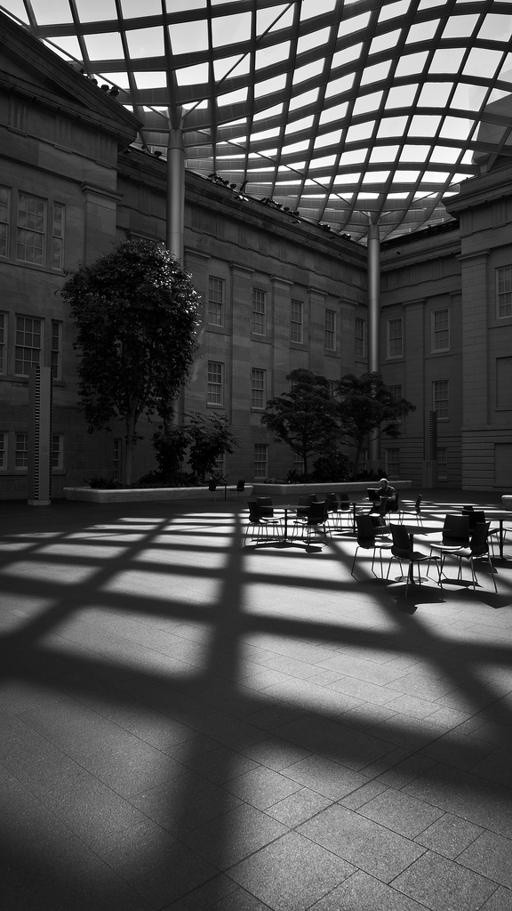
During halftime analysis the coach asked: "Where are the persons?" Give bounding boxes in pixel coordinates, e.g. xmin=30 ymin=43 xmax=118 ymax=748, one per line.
xmin=375 ymin=478 xmax=396 ymax=517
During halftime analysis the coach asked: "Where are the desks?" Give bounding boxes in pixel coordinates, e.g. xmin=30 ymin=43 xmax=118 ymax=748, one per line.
xmin=474 ymin=510 xmax=512 ymax=567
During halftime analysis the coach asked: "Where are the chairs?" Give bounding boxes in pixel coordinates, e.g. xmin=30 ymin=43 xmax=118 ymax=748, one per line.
xmin=426 ymin=512 xmax=469 ymax=581
xmin=462 ymin=509 xmax=496 ymax=558
xmin=227 ymin=479 xmax=247 ymax=505
xmin=385 ymin=522 xmax=444 ymax=603
xmin=439 ymin=522 xmax=498 ymax=593
xmin=243 ymin=487 xmax=422 ymax=555
xmin=350 ymin=515 xmax=393 ymax=581
xmin=208 ymin=479 xmax=224 ymax=503
